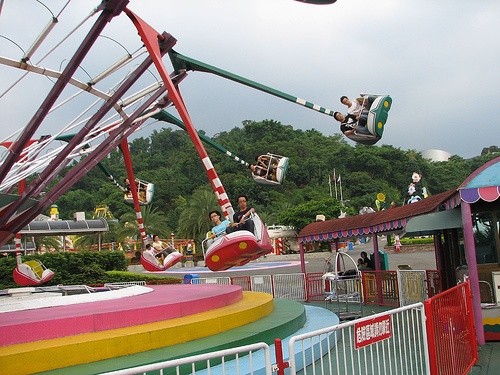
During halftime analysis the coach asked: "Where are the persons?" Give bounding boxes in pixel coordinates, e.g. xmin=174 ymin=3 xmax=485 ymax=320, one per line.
xmin=248 ymin=155 xmax=280 ymax=181
xmin=123 ymin=178 xmax=147 ymax=202
xmin=146 ymin=234 xmax=167 ymax=265
xmin=206 ymin=194 xmax=255 ymax=247
xmin=357 ymin=250 xmax=374 ymax=268
xmin=334 ymin=96 xmax=378 ymax=136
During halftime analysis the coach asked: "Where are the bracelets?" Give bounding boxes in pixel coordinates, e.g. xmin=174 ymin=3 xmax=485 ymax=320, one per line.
xmin=243 ymin=215 xmax=246 ymax=219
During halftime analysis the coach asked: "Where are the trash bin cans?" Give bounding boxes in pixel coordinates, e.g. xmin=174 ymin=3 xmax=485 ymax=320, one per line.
xmin=349 ymin=242 xmax=353 ymax=250
